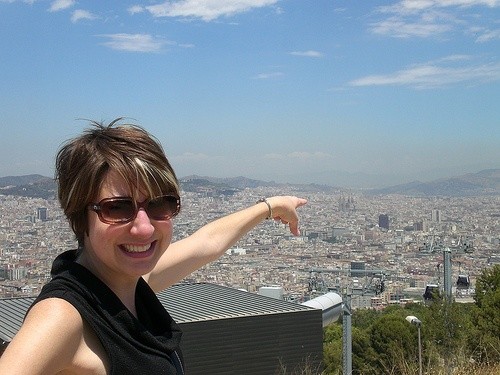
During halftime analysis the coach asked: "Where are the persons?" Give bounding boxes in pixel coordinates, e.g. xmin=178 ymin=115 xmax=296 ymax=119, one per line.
xmin=0 ymin=115 xmax=308 ymax=375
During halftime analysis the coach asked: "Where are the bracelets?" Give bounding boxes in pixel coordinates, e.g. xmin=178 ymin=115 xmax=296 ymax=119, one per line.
xmin=256 ymin=197 xmax=272 ymax=220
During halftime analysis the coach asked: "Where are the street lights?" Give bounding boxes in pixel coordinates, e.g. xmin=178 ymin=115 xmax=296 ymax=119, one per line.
xmin=405 ymin=315 xmax=423 ymax=374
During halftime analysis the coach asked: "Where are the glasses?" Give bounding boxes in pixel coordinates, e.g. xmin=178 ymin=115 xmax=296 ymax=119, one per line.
xmin=86 ymin=193 xmax=180 ymax=225
